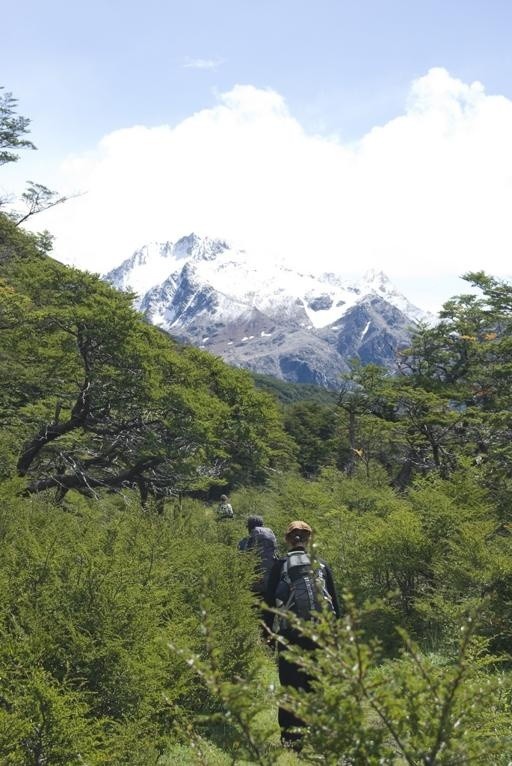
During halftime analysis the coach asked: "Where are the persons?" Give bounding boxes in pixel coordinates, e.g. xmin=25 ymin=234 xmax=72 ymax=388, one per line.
xmin=238 ymin=515 xmax=280 ymax=599
xmin=261 ymin=520 xmax=341 ymax=752
xmin=216 ymin=494 xmax=233 ymax=522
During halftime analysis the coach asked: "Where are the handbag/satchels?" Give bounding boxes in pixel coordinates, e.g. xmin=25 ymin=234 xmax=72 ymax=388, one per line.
xmin=249 ymin=526 xmax=277 ymax=572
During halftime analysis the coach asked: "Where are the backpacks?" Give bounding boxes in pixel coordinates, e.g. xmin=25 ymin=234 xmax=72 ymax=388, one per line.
xmin=219 ymin=503 xmax=232 ymax=519
xmin=278 ymin=547 xmax=338 ymax=642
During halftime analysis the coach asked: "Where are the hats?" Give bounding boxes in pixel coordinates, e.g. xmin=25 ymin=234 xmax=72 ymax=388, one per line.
xmin=244 ymin=515 xmax=265 ymax=529
xmin=285 ymin=519 xmax=313 ymax=537
xmin=221 ymin=494 xmax=228 ymax=502
